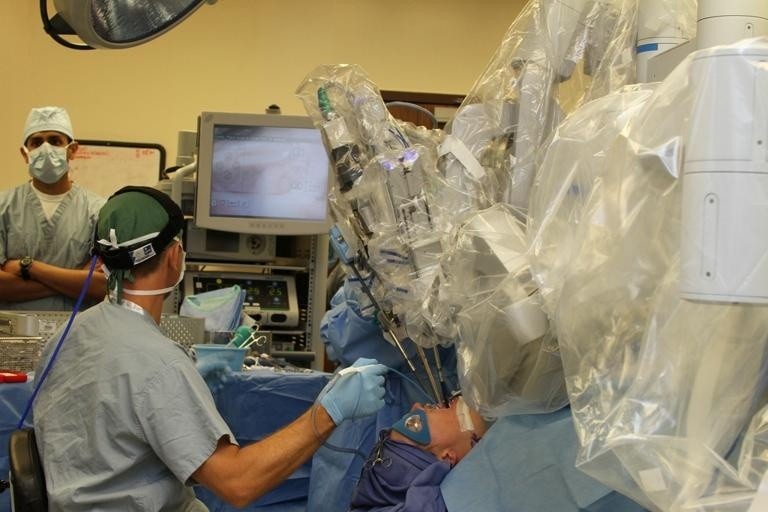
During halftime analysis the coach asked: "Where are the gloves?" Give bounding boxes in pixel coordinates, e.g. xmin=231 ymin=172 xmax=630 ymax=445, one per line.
xmin=194 ymin=351 xmax=241 ymax=396
xmin=316 ymin=357 xmax=390 ymax=428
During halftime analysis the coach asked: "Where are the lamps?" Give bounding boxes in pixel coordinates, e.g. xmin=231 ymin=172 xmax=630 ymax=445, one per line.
xmin=38 ymin=0 xmax=217 ymax=53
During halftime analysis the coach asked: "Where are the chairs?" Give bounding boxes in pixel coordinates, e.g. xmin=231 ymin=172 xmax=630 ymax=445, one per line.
xmin=8 ymin=426 xmax=48 ymax=512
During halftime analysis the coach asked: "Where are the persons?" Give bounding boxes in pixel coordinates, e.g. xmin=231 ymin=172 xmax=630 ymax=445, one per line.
xmin=353 ymin=391 xmax=487 ymax=512
xmin=305 ymin=272 xmax=459 ymax=512
xmin=32 ymin=186 xmax=389 ymax=512
xmin=0 ymin=105 xmax=107 ymax=312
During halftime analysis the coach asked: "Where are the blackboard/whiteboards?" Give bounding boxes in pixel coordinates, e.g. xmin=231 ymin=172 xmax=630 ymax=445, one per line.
xmin=68 ymin=140 xmax=166 ymax=200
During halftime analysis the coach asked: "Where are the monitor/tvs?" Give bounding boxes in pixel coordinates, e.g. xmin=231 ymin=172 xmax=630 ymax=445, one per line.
xmin=195 ymin=111 xmax=335 ymax=236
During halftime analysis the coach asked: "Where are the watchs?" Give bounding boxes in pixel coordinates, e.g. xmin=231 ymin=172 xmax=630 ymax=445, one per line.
xmin=21 ymin=255 xmax=35 ymax=279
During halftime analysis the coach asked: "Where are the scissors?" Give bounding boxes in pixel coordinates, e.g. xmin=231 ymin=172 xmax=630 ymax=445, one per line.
xmin=237 ymin=324 xmax=267 ymax=349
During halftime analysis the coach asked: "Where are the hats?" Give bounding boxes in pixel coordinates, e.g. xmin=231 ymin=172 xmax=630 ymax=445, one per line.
xmin=23 ymin=106 xmax=74 ymax=150
xmin=97 ymin=191 xmax=184 ymax=305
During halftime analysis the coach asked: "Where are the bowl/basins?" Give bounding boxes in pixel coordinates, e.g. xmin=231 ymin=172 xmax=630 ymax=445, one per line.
xmin=192 ymin=344 xmax=250 ymax=372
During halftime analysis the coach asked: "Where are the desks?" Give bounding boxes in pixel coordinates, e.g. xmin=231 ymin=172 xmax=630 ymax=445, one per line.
xmin=0 ymin=362 xmax=334 ymax=512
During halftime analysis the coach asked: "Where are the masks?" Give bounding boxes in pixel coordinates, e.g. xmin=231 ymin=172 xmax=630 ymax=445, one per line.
xmin=28 ymin=142 xmax=69 ymax=184
